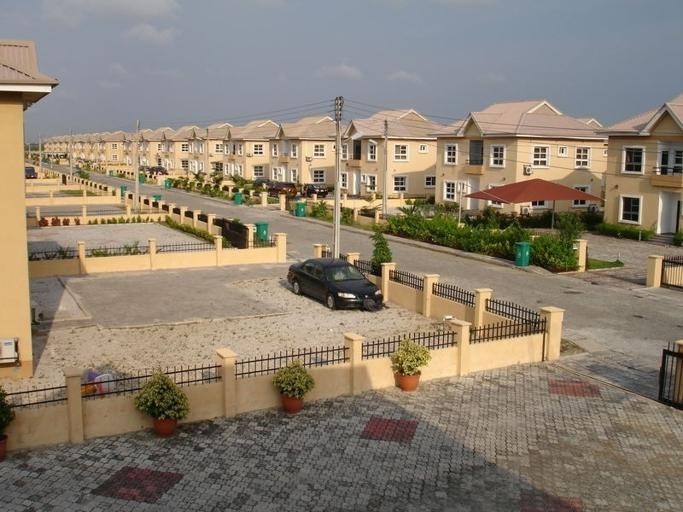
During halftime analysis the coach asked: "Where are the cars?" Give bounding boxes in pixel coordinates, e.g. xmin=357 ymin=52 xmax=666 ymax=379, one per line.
xmin=287 ymin=257 xmax=382 ymax=310
xmin=149 ymin=165 xmax=168 ymax=178
xmin=253 ymin=179 xmax=328 ymax=199
xmin=138 ymin=165 xmax=150 ymax=177
xmin=25 ymin=167 xmax=36 ymax=178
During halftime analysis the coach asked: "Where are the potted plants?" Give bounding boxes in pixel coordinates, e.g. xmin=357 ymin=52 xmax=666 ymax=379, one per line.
xmin=389 ymin=338 xmax=432 ymax=393
xmin=270 ymin=361 xmax=316 ymax=414
xmin=0 ymin=386 xmax=17 ymax=463
xmin=134 ymin=371 xmax=190 ymax=437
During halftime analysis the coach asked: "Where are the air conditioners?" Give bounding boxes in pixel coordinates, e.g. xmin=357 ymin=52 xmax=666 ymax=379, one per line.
xmin=524 ymin=164 xmax=533 ymax=176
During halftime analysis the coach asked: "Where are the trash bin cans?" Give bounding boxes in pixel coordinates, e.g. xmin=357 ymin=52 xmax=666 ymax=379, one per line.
xmin=139 ymin=176 xmax=145 ymax=183
xmin=153 ymin=195 xmax=161 ymax=201
xmin=256 ymin=223 xmax=268 ymax=241
xmin=296 ymin=203 xmax=305 ymax=216
xmin=109 ymin=171 xmax=113 ymax=175
xmin=515 ymin=242 xmax=531 ymax=266
xmin=165 ymin=180 xmax=171 ymax=188
xmin=121 ymin=185 xmax=126 ymax=195
xmin=235 ymin=192 xmax=242 ymax=205
